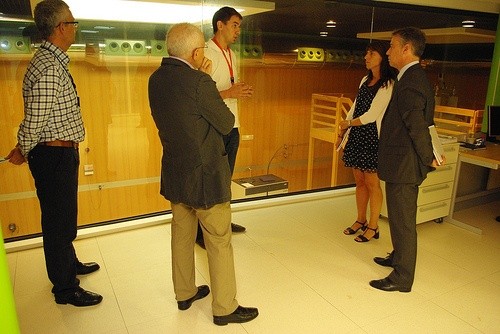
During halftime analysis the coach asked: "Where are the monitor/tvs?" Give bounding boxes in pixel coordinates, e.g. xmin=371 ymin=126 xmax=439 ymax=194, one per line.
xmin=487 ymin=106 xmax=500 ymax=144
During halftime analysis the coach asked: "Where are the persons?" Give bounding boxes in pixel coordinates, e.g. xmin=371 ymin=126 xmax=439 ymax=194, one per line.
xmin=196 ymin=7 xmax=253 ymax=251
xmin=147 ymin=22 xmax=259 ymax=326
xmin=5 ymin=3 xmax=104 ymax=307
xmin=370 ymin=25 xmax=447 ymax=292
xmin=341 ymin=37 xmax=396 ymax=243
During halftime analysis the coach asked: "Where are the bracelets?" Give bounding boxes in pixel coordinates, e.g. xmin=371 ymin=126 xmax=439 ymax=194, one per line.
xmin=349 ymin=120 xmax=352 ymax=125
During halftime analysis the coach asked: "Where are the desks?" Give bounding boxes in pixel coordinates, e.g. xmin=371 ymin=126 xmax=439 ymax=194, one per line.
xmin=456 ymin=139 xmax=500 ymax=170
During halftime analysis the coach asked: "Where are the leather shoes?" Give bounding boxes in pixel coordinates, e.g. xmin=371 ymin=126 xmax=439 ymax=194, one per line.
xmin=79 ymin=261 xmax=100 ymax=275
xmin=54 ymin=287 xmax=103 ymax=307
xmin=374 ymin=252 xmax=396 ymax=267
xmin=370 ymin=277 xmax=410 ymax=292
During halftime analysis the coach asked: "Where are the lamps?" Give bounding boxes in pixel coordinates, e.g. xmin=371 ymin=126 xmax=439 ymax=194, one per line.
xmin=325 ymin=19 xmax=336 ymax=28
xmin=461 ymin=21 xmax=476 ymax=27
xmin=320 ymin=32 xmax=328 ymax=36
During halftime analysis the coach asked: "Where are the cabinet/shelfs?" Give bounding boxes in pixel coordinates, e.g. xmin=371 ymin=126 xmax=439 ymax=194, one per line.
xmin=376 ymin=136 xmax=462 ymax=225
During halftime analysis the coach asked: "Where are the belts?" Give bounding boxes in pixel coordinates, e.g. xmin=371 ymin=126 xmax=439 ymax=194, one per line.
xmin=39 ymin=139 xmax=79 ymax=148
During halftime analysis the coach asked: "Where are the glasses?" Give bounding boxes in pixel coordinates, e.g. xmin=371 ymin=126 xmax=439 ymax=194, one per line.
xmin=55 ymin=22 xmax=79 ymax=32
xmin=197 ymin=44 xmax=208 ymax=50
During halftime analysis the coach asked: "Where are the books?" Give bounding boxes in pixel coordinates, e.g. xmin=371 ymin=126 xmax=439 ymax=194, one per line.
xmin=336 ymin=126 xmax=352 ymax=151
xmin=428 ymin=124 xmax=444 ymax=165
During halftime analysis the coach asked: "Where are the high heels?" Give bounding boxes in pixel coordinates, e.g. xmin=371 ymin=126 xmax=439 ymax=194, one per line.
xmin=344 ymin=219 xmax=368 ymax=234
xmin=354 ymin=225 xmax=379 ymax=242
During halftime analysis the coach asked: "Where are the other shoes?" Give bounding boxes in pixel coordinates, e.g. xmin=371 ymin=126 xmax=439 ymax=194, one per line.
xmin=177 ymin=285 xmax=210 ymax=310
xmin=195 ymin=237 xmax=206 ymax=250
xmin=213 ymin=305 xmax=258 ymax=326
xmin=231 ymin=222 xmax=245 ymax=231
